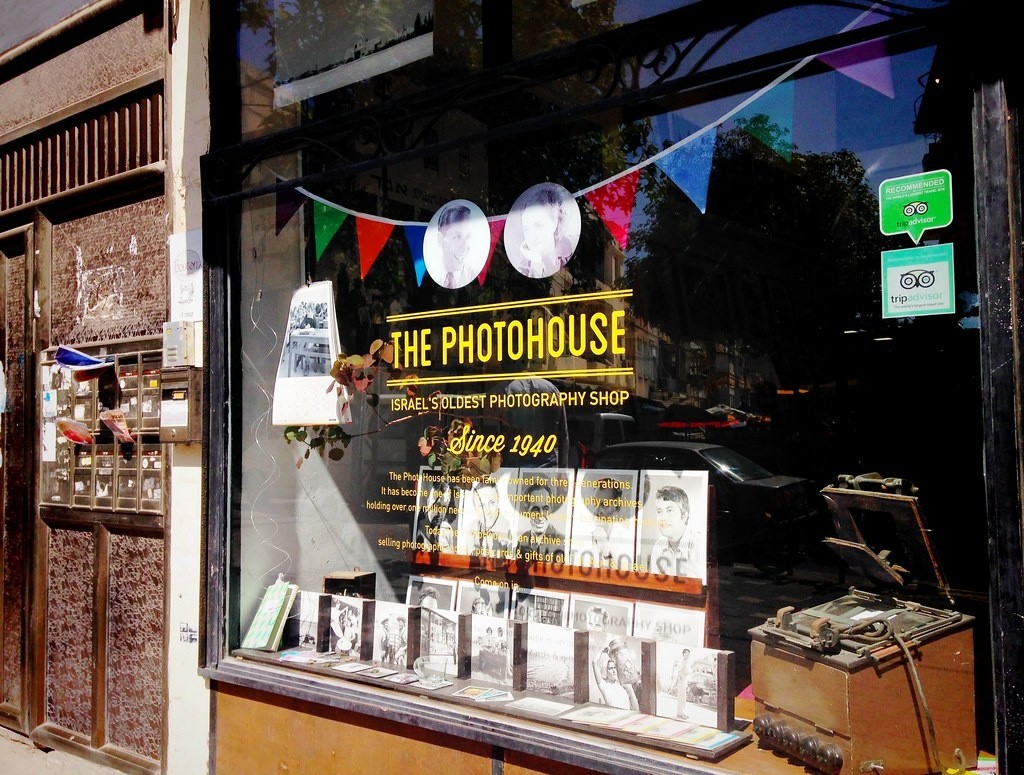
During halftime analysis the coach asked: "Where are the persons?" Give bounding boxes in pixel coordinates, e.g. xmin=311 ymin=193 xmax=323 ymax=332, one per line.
xmin=418 ymin=482 xmax=454 ymax=553
xmin=579 ymin=478 xmax=631 ymax=570
xmin=649 ymin=486 xmax=704 ymax=578
xmin=673 ymin=649 xmax=690 ymax=720
xmin=482 ymin=625 xmax=512 ymax=680
xmin=587 ymin=607 xmax=611 ymax=632
xmin=432 ymin=206 xmax=477 ymax=289
xmin=501 ymin=358 xmax=568 ymax=469
xmin=517 ymin=482 xmax=565 ymax=565
xmin=519 ymin=189 xmax=574 ymax=277
xmin=468 ymin=481 xmax=512 ymax=558
xmin=380 ymin=615 xmax=407 ymax=666
xmin=471 ymin=598 xmax=492 ymax=616
xmin=330 ymin=602 xmax=358 ymax=655
xmin=594 ymin=645 xmax=639 ymax=711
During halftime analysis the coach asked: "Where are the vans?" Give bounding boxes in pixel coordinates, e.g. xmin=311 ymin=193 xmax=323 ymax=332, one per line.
xmin=567 ymin=409 xmax=639 ymax=472
xmin=336 ymin=392 xmax=500 ymax=517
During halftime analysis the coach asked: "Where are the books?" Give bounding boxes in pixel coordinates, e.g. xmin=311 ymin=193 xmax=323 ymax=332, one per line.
xmin=240 ymin=581 xmax=299 ymax=652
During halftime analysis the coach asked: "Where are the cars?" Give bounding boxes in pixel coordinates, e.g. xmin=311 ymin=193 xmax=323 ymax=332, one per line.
xmin=583 ymin=440 xmax=819 ymax=535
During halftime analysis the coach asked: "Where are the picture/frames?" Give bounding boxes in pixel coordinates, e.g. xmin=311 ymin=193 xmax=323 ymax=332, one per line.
xmin=569 ymin=594 xmax=634 ymax=637
xmin=404 ymin=574 xmax=457 ymax=611
xmin=516 ymin=467 xmax=575 ymax=566
xmin=568 ymin=468 xmax=639 ymax=572
xmin=510 ymin=588 xmax=571 ymax=630
xmin=455 ymin=581 xmax=511 ymax=620
xmin=636 ymin=469 xmax=710 ymax=586
xmin=457 ymin=468 xmax=521 ymax=560
xmin=632 ymin=602 xmax=706 ymax=648
xmin=411 ymin=465 xmax=465 ymax=554
